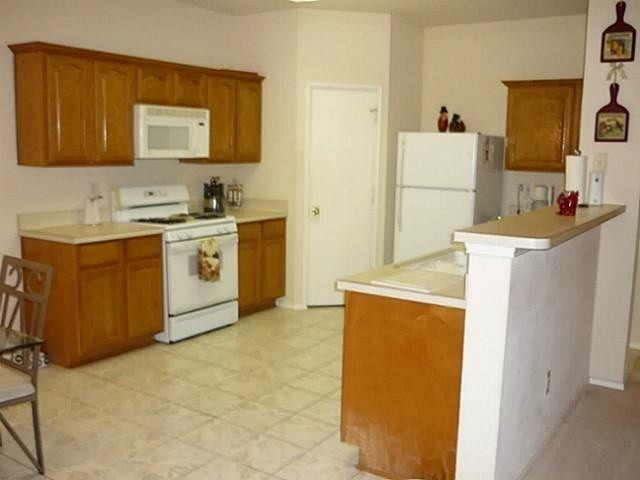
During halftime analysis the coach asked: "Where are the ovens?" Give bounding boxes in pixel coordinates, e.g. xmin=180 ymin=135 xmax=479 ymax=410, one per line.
xmin=153 ymin=232 xmax=240 ymax=343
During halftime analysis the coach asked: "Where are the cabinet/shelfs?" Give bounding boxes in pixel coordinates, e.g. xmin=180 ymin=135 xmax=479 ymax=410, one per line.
xmin=20 ymin=233 xmax=164 ymax=369
xmin=238 ymin=218 xmax=286 ymax=318
xmin=500 ymin=78 xmax=583 ymax=173
xmin=7 ymin=42 xmax=266 ymax=166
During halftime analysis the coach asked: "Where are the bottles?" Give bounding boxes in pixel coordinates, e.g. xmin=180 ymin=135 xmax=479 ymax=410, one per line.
xmin=564 ymin=155 xmax=587 ymax=205
xmin=530 ymin=186 xmax=548 ymax=211
xmin=438 ymin=106 xmax=466 ymax=132
xmin=228 ymin=184 xmax=243 ymax=205
xmin=86 ymin=193 xmax=107 ymax=226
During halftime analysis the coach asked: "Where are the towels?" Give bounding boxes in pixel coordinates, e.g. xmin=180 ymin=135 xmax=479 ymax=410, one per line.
xmin=198 ymin=238 xmax=223 ymax=283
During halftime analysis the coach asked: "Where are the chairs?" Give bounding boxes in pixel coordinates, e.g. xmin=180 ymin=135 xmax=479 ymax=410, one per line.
xmin=0 ymin=254 xmax=54 ymax=474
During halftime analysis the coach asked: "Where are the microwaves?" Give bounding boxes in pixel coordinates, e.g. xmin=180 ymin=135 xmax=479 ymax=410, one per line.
xmin=133 ymin=104 xmax=210 ymax=159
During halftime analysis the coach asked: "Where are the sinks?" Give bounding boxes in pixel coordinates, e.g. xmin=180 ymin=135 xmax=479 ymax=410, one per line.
xmin=393 ymin=247 xmax=470 ymax=277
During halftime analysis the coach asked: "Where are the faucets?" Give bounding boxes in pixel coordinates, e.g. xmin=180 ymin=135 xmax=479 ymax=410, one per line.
xmin=536 ymin=183 xmax=557 ymax=209
xmin=515 ymin=179 xmax=527 ymax=216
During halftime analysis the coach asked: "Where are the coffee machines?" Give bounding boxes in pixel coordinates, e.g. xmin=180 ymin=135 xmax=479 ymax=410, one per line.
xmin=205 ymin=176 xmax=225 ymax=214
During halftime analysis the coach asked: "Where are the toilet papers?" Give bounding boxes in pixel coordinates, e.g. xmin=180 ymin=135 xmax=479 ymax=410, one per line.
xmin=562 ymin=147 xmax=588 ymax=207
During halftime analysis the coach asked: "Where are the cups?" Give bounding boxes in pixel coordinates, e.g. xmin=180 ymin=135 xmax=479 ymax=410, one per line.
xmin=557 ymin=191 xmax=580 ymax=216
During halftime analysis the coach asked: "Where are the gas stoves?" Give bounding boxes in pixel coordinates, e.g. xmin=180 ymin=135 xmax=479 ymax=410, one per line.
xmin=129 ymin=212 xmax=238 ymax=242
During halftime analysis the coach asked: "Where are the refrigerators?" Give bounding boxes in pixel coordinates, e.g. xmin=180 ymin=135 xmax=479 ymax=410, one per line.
xmin=395 ymin=131 xmax=505 ymax=261
